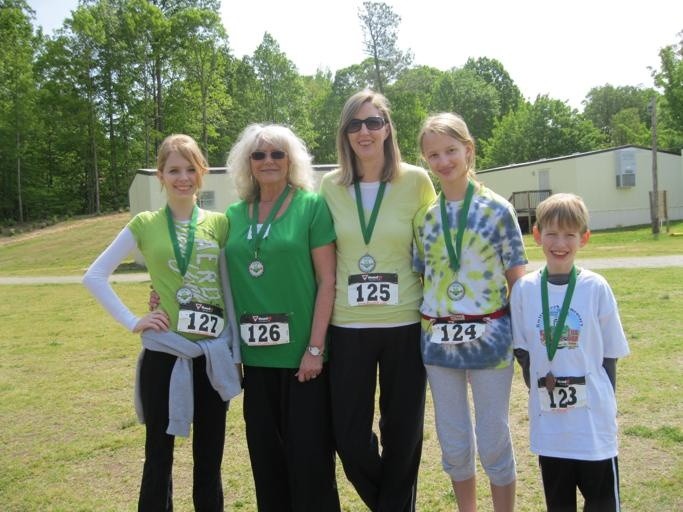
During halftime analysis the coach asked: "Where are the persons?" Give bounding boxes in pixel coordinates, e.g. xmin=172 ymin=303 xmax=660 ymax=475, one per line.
xmin=506 ymin=192 xmax=630 ymax=512
xmin=406 ymin=112 xmax=531 ymax=512
xmin=78 ymin=133 xmax=245 ymax=511
xmin=148 ymin=121 xmax=341 ymax=511
xmin=316 ymin=89 xmax=438 ymax=511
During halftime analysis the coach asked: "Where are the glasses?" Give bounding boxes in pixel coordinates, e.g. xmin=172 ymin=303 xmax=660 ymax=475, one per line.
xmin=250 ymin=152 xmax=287 ymax=160
xmin=345 ymin=116 xmax=384 ymax=133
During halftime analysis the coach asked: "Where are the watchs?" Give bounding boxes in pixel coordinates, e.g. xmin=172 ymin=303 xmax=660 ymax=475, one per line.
xmin=305 ymin=345 xmax=325 ymax=359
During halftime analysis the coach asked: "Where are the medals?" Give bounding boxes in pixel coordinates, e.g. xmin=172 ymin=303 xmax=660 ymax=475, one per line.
xmin=446 ymin=280 xmax=466 ymax=302
xmin=175 ymin=285 xmax=193 ymax=307
xmin=357 ymin=253 xmax=375 ymax=274
xmin=544 ymin=371 xmax=557 ymax=393
xmin=247 ymin=260 xmax=264 ymax=279
